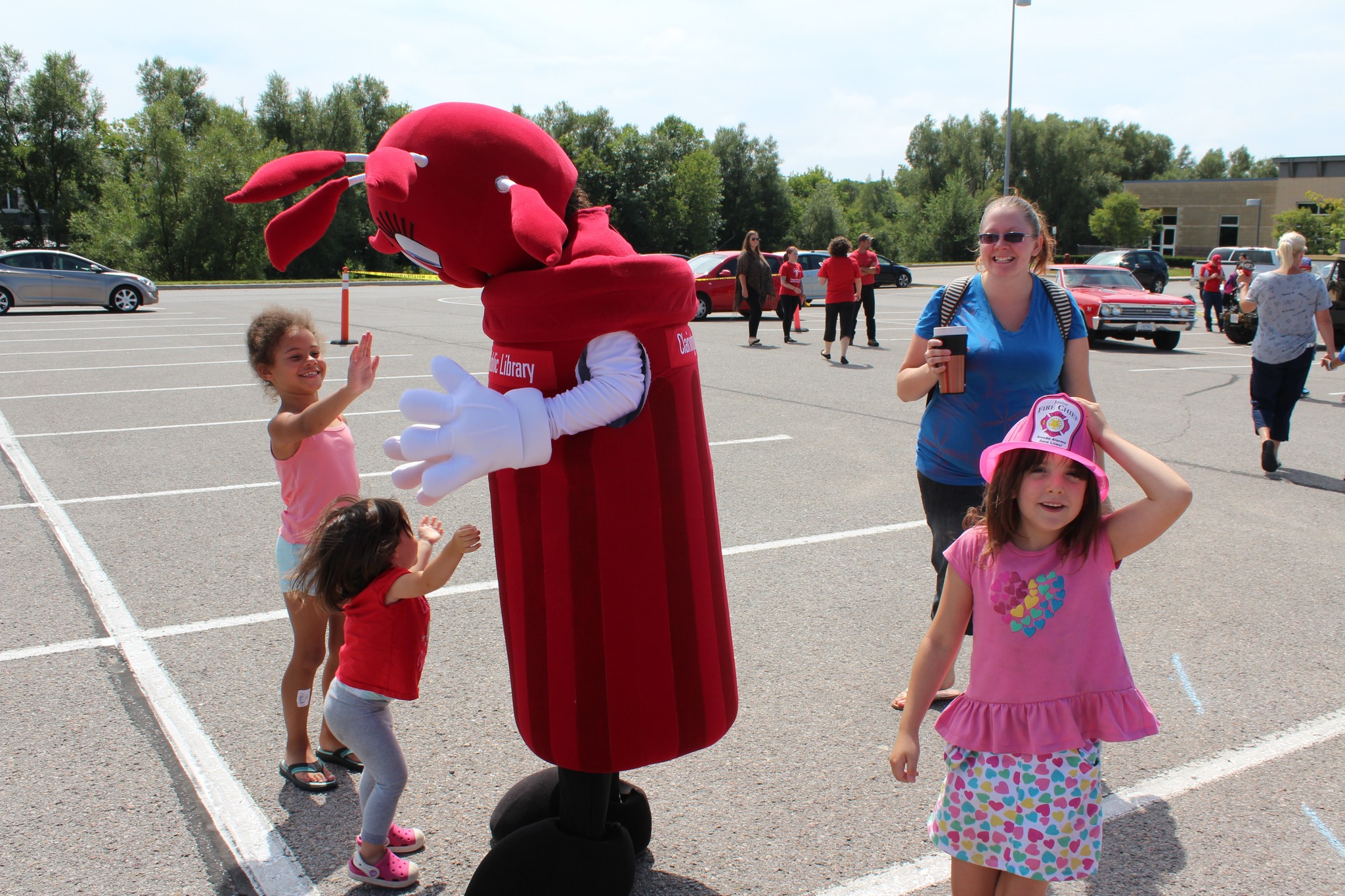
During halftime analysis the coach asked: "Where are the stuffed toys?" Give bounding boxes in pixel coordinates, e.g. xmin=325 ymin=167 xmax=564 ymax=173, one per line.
xmin=225 ymin=103 xmax=739 ymax=896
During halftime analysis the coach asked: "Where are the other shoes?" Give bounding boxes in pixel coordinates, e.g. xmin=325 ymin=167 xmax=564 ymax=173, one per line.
xmin=1207 ymin=328 xmax=1212 ymax=331
xmin=1301 ymin=388 xmax=1310 ymax=396
xmin=752 ymin=343 xmax=762 ymax=346
xmin=748 ymin=338 xmax=760 ymax=346
xmin=784 ymin=336 xmax=790 ymax=343
xmin=1261 ymin=440 xmax=1281 ymax=472
xmin=788 ymin=339 xmax=798 ymax=342
xmin=1341 ymin=395 xmax=1345 ymax=403
xmin=848 ymin=339 xmax=853 ymax=345
xmin=1220 ymin=329 xmax=1224 ymax=332
xmin=868 ymin=339 xmax=879 ymax=346
xmin=841 ymin=356 xmax=849 ymax=364
xmin=821 ymin=349 xmax=831 ymax=359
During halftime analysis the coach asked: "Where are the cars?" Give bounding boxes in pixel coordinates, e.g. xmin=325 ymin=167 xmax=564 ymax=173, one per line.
xmin=771 ymin=250 xmax=851 ymax=306
xmin=0 ymin=249 xmax=159 ymax=317
xmin=1218 ymin=252 xmax=1345 ymax=351
xmin=686 ymin=250 xmax=801 ymax=322
xmin=1027 ymin=263 xmax=1198 ymax=350
xmin=847 ymin=250 xmax=912 ymax=289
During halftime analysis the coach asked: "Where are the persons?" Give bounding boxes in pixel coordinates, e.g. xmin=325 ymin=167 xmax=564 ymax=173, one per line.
xmin=888 ymin=392 xmax=1193 ymax=896
xmin=245 ymin=310 xmax=379 ymax=791
xmin=779 ymin=246 xmax=804 ymax=343
xmin=1199 ymin=253 xmax=1249 ymax=333
xmin=848 ymin=232 xmax=880 ymax=346
xmin=734 ymin=231 xmax=776 ymax=347
xmin=1236 ymin=232 xmax=1336 ymax=472
xmin=290 ymin=494 xmax=483 ymax=889
xmin=1299 ymin=257 xmax=1313 ymax=397
xmin=818 ymin=237 xmax=862 ymax=365
xmin=893 ymin=196 xmax=1111 ymax=710
xmin=1321 ymin=345 xmax=1345 ymax=372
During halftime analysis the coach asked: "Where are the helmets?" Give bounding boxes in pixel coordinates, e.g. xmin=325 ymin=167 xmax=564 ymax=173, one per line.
xmin=979 ymin=392 xmax=1109 ymax=502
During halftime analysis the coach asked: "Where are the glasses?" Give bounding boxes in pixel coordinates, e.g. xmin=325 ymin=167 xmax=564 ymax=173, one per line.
xmin=979 ymin=232 xmax=1033 ymax=245
xmin=750 ymin=237 xmax=759 ymax=241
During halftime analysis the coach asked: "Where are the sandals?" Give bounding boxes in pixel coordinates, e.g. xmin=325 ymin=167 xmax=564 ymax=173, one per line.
xmin=347 ymin=848 xmax=419 ymax=888
xmin=356 ymin=822 xmax=425 ymax=853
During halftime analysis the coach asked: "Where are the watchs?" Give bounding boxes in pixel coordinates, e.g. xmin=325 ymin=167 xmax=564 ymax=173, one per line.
xmin=1238 ymin=282 xmax=1249 ymax=288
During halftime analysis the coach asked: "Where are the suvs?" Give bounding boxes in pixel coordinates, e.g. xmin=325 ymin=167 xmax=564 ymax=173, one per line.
xmin=1077 ymin=248 xmax=1169 ymax=295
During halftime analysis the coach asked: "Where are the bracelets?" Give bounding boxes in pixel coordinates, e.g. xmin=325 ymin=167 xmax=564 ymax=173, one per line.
xmin=794 ymin=287 xmax=796 ymax=290
xmin=1328 ymin=362 xmax=1337 ymax=370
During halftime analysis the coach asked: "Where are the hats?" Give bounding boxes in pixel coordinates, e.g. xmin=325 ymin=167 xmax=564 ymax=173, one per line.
xmin=858 ymin=233 xmax=874 ymax=242
xmin=1298 ymin=257 xmax=1312 ymax=269
xmin=1213 ymin=254 xmax=1223 ymax=265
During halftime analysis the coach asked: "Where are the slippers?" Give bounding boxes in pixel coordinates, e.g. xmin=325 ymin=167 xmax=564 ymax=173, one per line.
xmin=315 ymin=745 xmax=364 ymax=770
xmin=891 ymin=688 xmax=960 ymax=709
xmin=280 ymin=756 xmax=339 ymax=791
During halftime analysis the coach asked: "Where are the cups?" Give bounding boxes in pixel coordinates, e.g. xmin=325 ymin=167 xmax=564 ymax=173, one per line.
xmin=933 ymin=327 xmax=969 ymax=395
xmin=1242 ymin=262 xmax=1255 ymax=276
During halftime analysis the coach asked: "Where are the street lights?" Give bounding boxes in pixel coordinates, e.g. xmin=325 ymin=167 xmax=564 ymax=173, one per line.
xmin=1246 ymin=198 xmax=1261 ymax=247
xmin=1002 ymin=0 xmax=1033 ymax=197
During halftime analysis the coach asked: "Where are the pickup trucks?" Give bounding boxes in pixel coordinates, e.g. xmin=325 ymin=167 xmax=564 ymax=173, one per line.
xmin=1189 ymin=246 xmax=1281 ymax=300
